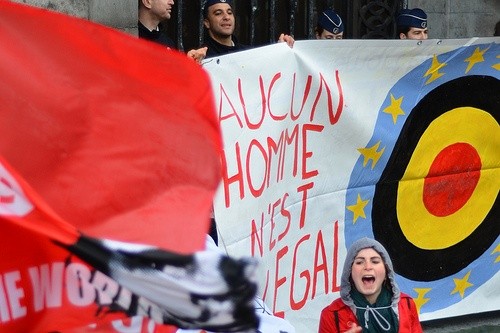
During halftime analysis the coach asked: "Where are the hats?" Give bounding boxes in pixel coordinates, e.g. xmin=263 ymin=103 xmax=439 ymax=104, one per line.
xmin=398 ymin=8 xmax=428 ymax=29
xmin=203 ymin=0 xmax=228 ymax=12
xmin=319 ymin=10 xmax=344 ymax=34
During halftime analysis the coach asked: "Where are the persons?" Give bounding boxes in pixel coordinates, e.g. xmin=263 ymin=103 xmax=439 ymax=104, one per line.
xmin=395 ymin=8 xmax=429 ymax=40
xmin=315 ymin=10 xmax=344 ymax=40
xmin=138 ymin=0 xmax=208 ymax=62
xmin=201 ymin=0 xmax=294 ymax=59
xmin=318 ymin=238 xmax=423 ymax=333
